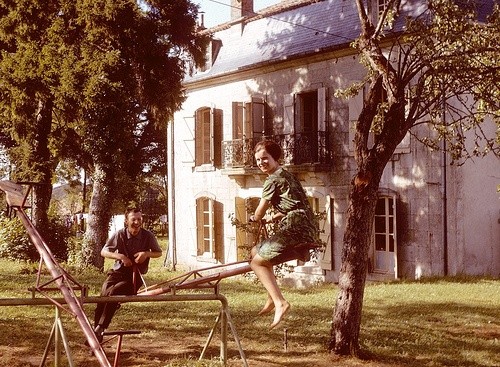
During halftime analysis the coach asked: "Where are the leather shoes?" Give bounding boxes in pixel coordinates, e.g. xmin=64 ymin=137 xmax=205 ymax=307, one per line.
xmin=85 ymin=333 xmax=102 ymax=346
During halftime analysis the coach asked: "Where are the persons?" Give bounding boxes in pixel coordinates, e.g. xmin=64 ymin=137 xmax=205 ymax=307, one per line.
xmin=84 ymin=207 xmax=163 ymax=349
xmin=249 ymin=140 xmax=313 ymax=328
